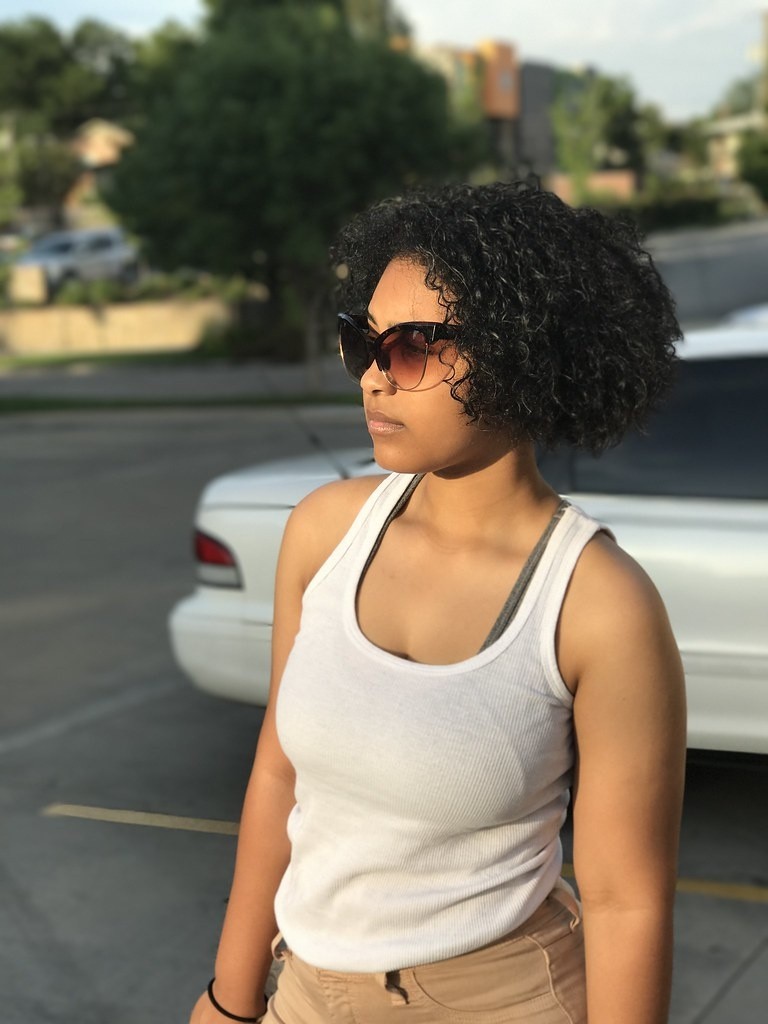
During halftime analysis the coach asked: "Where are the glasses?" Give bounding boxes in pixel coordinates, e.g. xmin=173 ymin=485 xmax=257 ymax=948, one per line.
xmin=336 ymin=314 xmax=464 ymax=390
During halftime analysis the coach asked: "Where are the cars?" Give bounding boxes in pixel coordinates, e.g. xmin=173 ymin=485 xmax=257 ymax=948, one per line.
xmin=6 ymin=227 xmax=133 ymax=304
xmin=159 ymin=315 xmax=768 ymax=759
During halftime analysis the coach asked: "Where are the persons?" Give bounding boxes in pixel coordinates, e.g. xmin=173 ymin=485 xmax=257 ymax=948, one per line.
xmin=186 ymin=174 xmax=688 ymax=1024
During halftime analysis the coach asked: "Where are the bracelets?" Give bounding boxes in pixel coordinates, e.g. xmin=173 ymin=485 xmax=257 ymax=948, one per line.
xmin=207 ymin=977 xmax=269 ymax=1023
